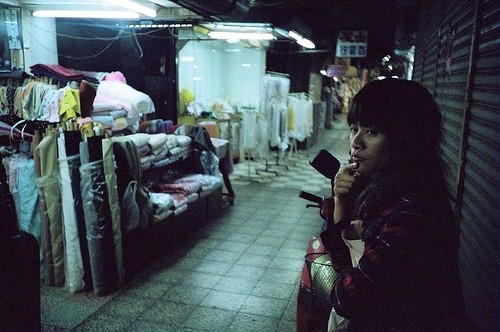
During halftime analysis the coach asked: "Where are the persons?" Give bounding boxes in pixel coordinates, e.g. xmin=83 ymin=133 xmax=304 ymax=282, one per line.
xmin=297 ymin=77 xmax=461 ymax=332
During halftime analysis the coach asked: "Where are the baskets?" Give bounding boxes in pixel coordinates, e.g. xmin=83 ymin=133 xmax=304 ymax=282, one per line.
xmin=305 ymin=252 xmax=360 ymax=309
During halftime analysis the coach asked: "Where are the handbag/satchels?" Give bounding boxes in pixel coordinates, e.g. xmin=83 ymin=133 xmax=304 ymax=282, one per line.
xmin=1 ymin=181 xmax=19 ymax=234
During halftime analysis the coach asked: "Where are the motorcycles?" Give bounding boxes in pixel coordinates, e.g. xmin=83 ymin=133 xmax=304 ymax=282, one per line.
xmin=299 ymin=149 xmax=482 ymax=332
